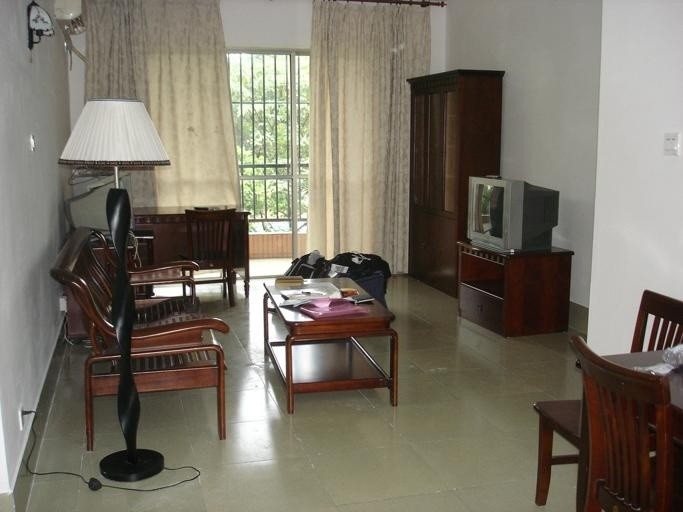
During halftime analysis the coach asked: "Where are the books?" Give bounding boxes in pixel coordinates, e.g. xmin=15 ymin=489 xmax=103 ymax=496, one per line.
xmin=275 ymin=276 xmax=303 ymax=288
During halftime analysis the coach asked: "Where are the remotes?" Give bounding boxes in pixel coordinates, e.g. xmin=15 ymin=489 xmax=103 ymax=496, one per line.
xmin=485 ymin=175 xmax=502 ymax=179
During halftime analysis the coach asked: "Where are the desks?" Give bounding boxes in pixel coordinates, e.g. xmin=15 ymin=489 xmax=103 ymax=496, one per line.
xmin=132 ymin=206 xmax=251 ymax=299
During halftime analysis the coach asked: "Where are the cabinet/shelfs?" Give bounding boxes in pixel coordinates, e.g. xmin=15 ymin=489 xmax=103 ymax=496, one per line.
xmin=405 ymin=68 xmax=506 ymax=299
xmin=456 ymin=241 xmax=574 ymax=338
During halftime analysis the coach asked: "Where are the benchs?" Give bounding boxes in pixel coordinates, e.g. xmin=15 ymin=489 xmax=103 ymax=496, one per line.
xmin=51 ymin=224 xmax=228 ymax=453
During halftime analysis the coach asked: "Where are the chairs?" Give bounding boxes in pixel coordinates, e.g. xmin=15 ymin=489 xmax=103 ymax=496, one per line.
xmin=185 ymin=209 xmax=237 ymax=306
xmin=532 ymin=289 xmax=683 ymax=512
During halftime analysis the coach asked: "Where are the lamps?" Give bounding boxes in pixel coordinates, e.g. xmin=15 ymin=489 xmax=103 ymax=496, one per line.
xmin=53 ymin=100 xmax=176 ymax=484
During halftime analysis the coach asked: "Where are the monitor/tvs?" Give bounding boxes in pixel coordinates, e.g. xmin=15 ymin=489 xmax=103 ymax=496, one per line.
xmin=467 ymin=176 xmax=561 ymax=256
